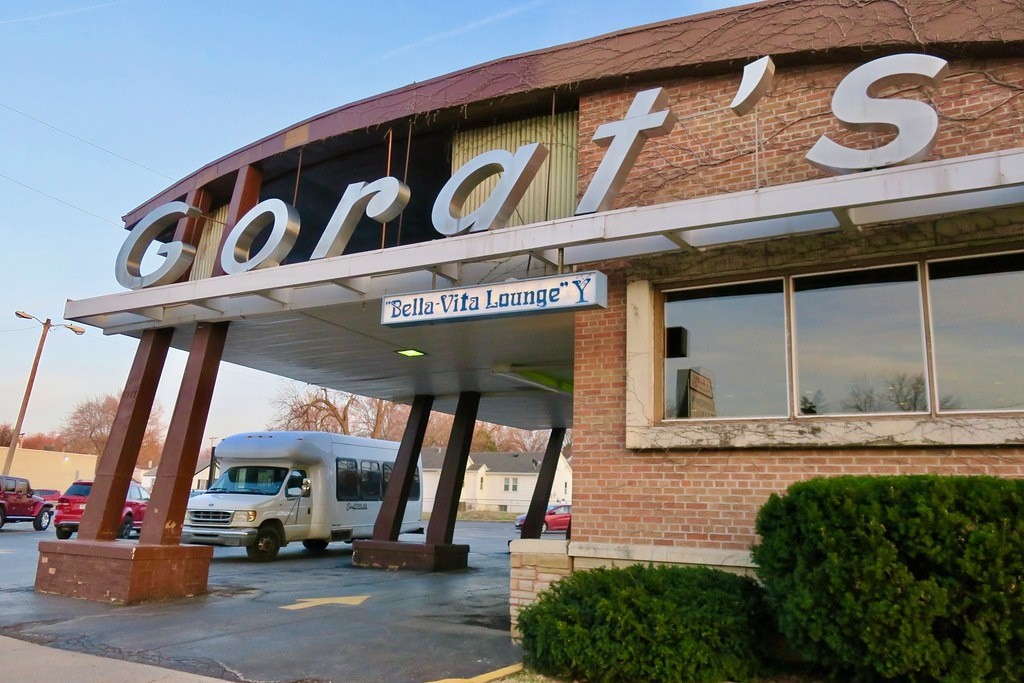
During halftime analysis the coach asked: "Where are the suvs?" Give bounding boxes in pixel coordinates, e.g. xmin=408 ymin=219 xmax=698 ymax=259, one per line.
xmin=54 ymin=480 xmax=151 ymax=539
xmin=31 ymin=488 xmax=62 ymax=517
xmin=0 ymin=475 xmax=53 ymax=531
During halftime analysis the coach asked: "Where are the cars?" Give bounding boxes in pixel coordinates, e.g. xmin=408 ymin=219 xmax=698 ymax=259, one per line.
xmin=514 ymin=504 xmax=572 ymax=533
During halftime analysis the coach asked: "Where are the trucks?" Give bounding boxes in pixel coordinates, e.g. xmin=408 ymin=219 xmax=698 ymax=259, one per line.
xmin=182 ymin=429 xmax=425 ymax=561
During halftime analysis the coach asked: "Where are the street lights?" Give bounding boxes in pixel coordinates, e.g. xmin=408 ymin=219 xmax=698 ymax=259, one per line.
xmin=2 ymin=311 xmax=86 ymax=478
